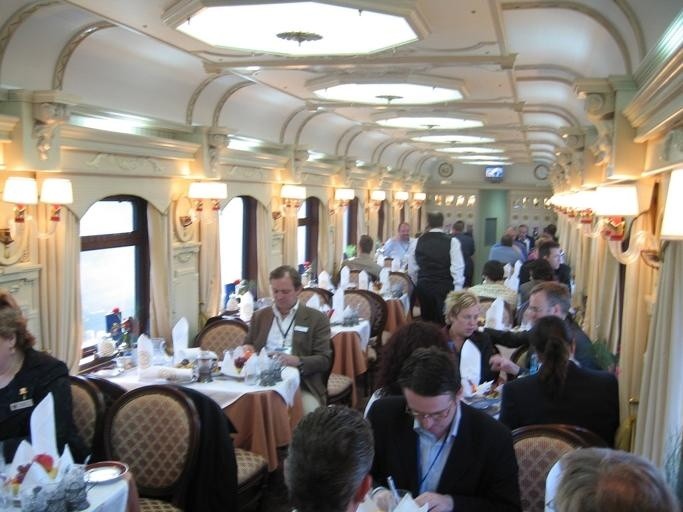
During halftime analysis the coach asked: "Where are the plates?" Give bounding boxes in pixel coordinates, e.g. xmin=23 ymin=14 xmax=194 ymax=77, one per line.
xmin=82 ymin=460 xmax=128 ymax=485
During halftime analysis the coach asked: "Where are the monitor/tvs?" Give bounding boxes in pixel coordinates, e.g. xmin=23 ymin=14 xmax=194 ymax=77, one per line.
xmin=485 ymin=165 xmax=505 ymax=184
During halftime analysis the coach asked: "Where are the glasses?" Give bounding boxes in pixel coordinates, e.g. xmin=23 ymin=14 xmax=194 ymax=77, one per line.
xmin=404 ymin=404 xmax=452 ymax=418
xmin=529 ymin=303 xmax=555 ymax=313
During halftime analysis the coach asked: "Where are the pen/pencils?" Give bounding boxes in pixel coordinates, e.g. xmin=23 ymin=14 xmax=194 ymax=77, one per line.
xmin=387 ymin=475 xmax=401 ymax=503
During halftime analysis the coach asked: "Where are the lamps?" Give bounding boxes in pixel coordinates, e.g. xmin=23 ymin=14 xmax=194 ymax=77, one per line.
xmin=544 ymin=181 xmax=638 ymax=243
xmin=658 ymin=164 xmax=682 ymax=243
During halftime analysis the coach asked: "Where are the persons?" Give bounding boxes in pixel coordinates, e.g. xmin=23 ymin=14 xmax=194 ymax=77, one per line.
xmin=363 ymin=346 xmax=522 ymax=512
xmin=544 ymin=446 xmax=682 ymax=512
xmin=382 ymin=212 xmax=475 ymax=325
xmin=282 ymin=405 xmax=377 ymax=511
xmin=367 ymin=282 xmax=619 ymax=448
xmin=468 ymin=224 xmax=571 ymax=315
xmin=0 ymin=288 xmax=94 ymax=465
xmin=340 ymin=233 xmax=382 ymax=282
xmin=243 ymin=265 xmax=333 ymax=420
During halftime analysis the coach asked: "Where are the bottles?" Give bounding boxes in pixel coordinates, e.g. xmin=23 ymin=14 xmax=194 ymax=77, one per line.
xmin=115 ymin=350 xmax=133 ymax=370
